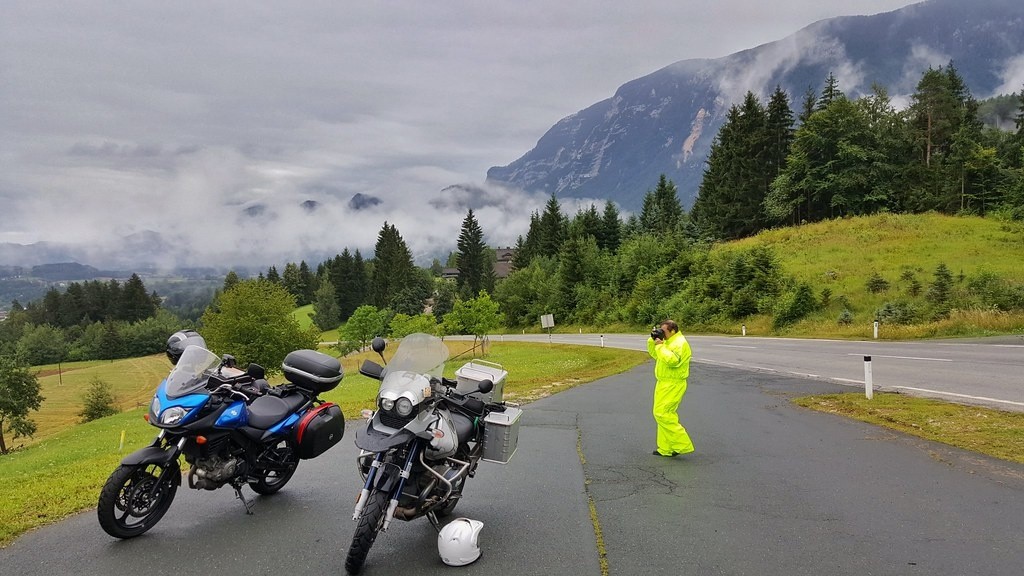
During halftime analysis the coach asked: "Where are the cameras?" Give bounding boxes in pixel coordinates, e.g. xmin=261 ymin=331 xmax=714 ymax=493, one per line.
xmin=651 ymin=328 xmax=665 ymax=340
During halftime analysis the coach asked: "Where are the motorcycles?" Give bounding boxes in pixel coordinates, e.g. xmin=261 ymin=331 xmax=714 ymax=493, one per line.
xmin=96 ymin=329 xmax=344 ymax=539
xmin=343 ymin=333 xmax=524 ymax=574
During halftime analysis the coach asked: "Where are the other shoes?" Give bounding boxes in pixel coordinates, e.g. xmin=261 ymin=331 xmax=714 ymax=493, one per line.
xmin=671 ymin=450 xmax=679 ymax=457
xmin=653 ymin=450 xmax=662 ymax=456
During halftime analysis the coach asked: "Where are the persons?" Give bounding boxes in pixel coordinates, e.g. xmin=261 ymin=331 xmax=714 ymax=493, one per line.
xmin=647 ymin=320 xmax=695 ymax=457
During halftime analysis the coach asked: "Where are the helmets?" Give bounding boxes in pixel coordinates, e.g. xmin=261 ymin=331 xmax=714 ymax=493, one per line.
xmin=165 ymin=329 xmax=208 ymax=365
xmin=437 ymin=517 xmax=484 ymax=567
